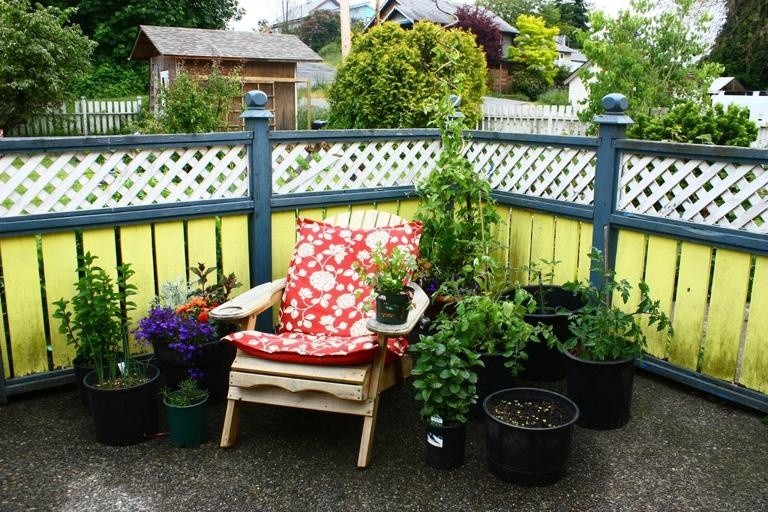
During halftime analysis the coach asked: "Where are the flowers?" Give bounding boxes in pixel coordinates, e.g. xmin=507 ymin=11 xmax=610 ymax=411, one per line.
xmin=131 ymin=261 xmax=249 ymax=392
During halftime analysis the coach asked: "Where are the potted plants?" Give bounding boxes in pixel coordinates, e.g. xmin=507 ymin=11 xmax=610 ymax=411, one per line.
xmin=163 ymin=380 xmax=209 ymax=448
xmin=71 ymin=275 xmax=159 ymax=445
xmin=497 ymin=247 xmax=675 ymax=430
xmin=355 ymin=238 xmax=421 ymax=324
xmin=399 ymin=0 xmax=536 ymax=471
xmin=53 ymin=250 xmax=126 ymax=406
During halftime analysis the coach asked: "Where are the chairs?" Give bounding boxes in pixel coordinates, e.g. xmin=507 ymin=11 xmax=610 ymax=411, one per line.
xmin=208 ymin=208 xmax=421 ymax=469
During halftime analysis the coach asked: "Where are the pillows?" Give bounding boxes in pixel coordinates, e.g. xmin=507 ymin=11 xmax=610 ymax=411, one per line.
xmin=277 ymin=215 xmax=425 ymax=351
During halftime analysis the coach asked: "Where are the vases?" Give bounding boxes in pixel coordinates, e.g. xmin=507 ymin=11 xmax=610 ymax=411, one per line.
xmin=482 ymin=386 xmax=580 ymax=488
